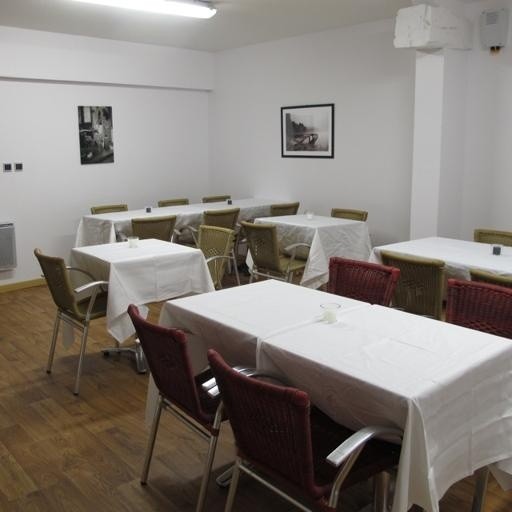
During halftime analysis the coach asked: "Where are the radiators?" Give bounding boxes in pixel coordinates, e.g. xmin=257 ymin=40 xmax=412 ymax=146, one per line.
xmin=0 ymin=223 xmax=16 ymax=272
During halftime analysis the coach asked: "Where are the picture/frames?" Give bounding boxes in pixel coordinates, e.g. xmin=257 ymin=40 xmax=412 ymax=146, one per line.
xmin=280 ymin=103 xmax=334 ymax=158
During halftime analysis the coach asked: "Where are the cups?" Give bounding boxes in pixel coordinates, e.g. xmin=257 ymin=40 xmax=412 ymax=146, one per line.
xmin=227 ymin=199 xmax=233 ymax=205
xmin=492 ymin=243 xmax=503 ymax=256
xmin=145 ymin=205 xmax=153 ymax=213
xmin=321 ymin=303 xmax=341 ymax=323
xmin=127 ymin=236 xmax=139 ymax=250
xmin=304 ymin=210 xmax=313 ymax=219
